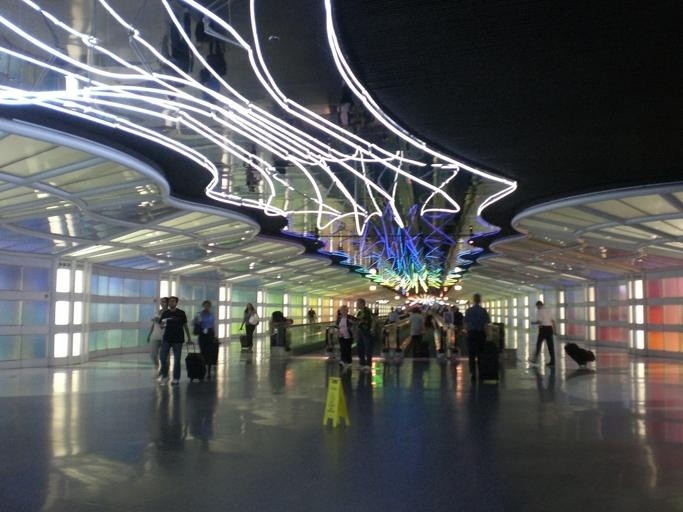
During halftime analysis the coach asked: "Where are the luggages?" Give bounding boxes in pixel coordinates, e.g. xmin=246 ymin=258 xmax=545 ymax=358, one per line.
xmin=270 ymin=334 xmax=291 ymax=344
xmin=477 ymin=341 xmax=500 ymax=371
xmin=239 ymin=335 xmax=249 ymax=348
xmin=212 ymin=338 xmax=219 ymax=362
xmin=184 ymin=351 xmax=207 ymax=382
xmin=564 ymin=341 xmax=596 ymax=367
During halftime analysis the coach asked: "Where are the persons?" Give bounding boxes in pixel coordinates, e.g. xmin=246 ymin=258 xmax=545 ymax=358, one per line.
xmin=240 ymin=302 xmax=259 ymax=347
xmin=307 ymin=308 xmax=317 ymax=324
xmin=532 ymin=366 xmax=558 ymax=428
xmin=527 ymin=300 xmax=558 ymax=366
xmin=145 ymin=297 xmax=168 ymax=377
xmin=333 ymin=293 xmax=493 ymax=379
xmin=190 ymin=301 xmax=215 ymax=380
xmin=156 ymin=386 xmax=184 ymax=452
xmin=157 ymin=296 xmax=190 ymax=385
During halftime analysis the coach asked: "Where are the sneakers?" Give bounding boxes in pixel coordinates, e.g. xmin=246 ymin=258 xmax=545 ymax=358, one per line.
xmin=151 ymin=371 xmax=178 ymax=386
xmin=528 ymin=356 xmax=556 ymax=367
xmin=337 ymin=361 xmax=373 ymax=370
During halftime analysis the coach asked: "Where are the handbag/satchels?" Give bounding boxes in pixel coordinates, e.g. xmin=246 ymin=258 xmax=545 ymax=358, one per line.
xmin=248 ymin=314 xmax=259 ymax=325
xmin=192 ymin=321 xmax=200 ymax=334
xmin=272 ymin=311 xmax=286 ymax=321
